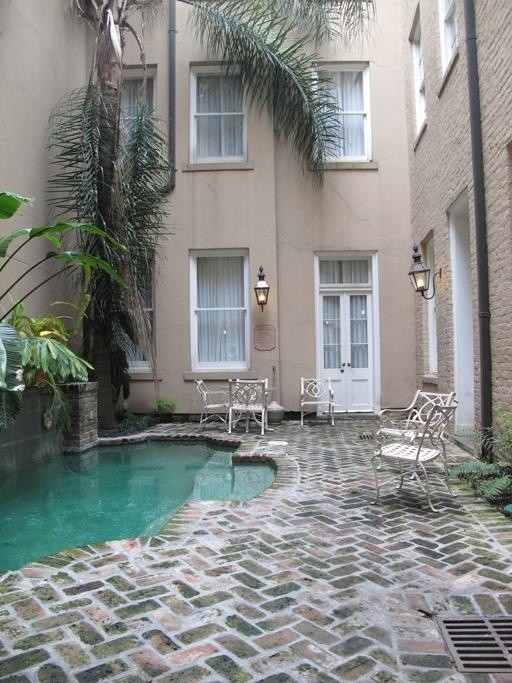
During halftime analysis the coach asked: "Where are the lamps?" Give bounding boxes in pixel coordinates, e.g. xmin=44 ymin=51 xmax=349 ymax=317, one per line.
xmin=253 ymin=265 xmax=270 ymax=312
xmin=409 ymin=242 xmax=445 ymax=300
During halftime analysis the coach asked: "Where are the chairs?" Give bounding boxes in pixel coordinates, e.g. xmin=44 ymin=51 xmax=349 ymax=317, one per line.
xmin=194 ymin=374 xmax=336 ymax=435
xmin=372 ymin=391 xmax=461 ymax=513
